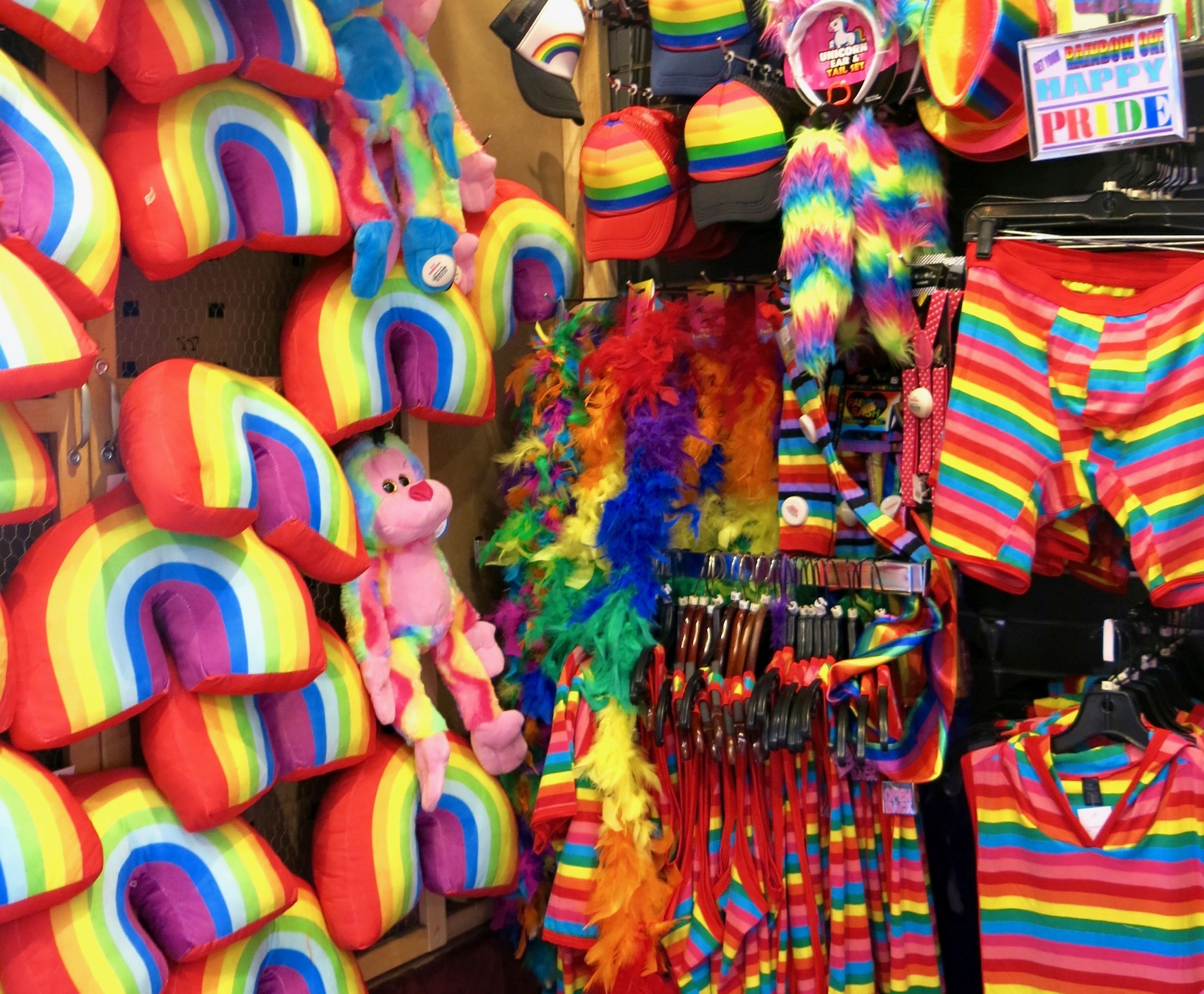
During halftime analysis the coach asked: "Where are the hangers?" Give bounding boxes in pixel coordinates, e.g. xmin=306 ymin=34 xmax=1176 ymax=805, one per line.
xmin=1051 ymin=607 xmax=1204 ymax=758
xmin=961 ymin=128 xmax=1204 ymax=252
xmin=626 ymin=549 xmax=903 ymax=762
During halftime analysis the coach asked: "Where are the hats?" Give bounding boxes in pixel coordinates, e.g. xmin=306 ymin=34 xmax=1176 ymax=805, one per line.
xmin=486 ymin=0 xmax=1081 ymax=262
